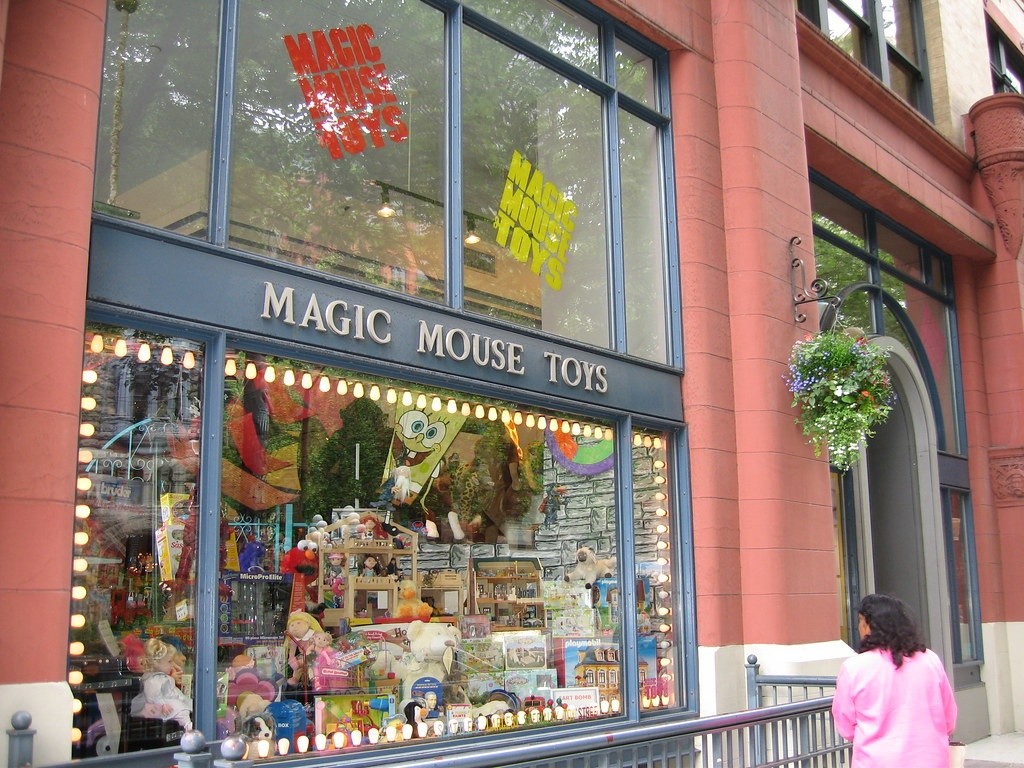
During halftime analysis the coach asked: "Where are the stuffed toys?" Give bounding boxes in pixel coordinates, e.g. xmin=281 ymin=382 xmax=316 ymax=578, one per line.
xmin=372 ymin=621 xmax=461 ymax=699
xmin=403 ymin=701 xmax=427 ymax=738
xmin=395 ymin=579 xmax=433 ymax=616
xmin=281 ymin=540 xmax=320 ymax=603
xmin=565 ymin=546 xmax=617 ymax=588
xmin=246 ymin=713 xmax=276 ymax=740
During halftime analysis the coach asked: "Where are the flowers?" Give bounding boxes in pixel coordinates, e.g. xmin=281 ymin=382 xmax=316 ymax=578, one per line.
xmin=778 ymin=313 xmax=899 ymax=474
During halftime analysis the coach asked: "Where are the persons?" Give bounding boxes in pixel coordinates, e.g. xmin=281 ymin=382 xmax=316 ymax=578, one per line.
xmin=326 ymin=553 xmax=348 ymax=597
xmin=458 ymin=419 xmax=524 ymax=544
xmin=362 ymin=517 xmax=388 ymax=542
xmin=360 ymin=554 xmax=379 ymax=577
xmin=141 ymin=639 xmax=192 ymax=730
xmin=831 ymin=594 xmax=957 ymax=768
xmin=283 ymin=611 xmax=332 ymax=690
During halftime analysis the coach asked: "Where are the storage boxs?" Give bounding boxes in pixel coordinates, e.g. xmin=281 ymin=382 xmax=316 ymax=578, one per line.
xmin=156 ymin=492 xmax=241 ymax=583
xmin=179 ymin=576 xmax=658 ymax=756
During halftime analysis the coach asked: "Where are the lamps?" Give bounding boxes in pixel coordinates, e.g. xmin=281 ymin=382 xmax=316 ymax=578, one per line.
xmin=463 ymin=215 xmax=481 ymax=245
xmin=377 ymin=186 xmax=396 ymax=219
